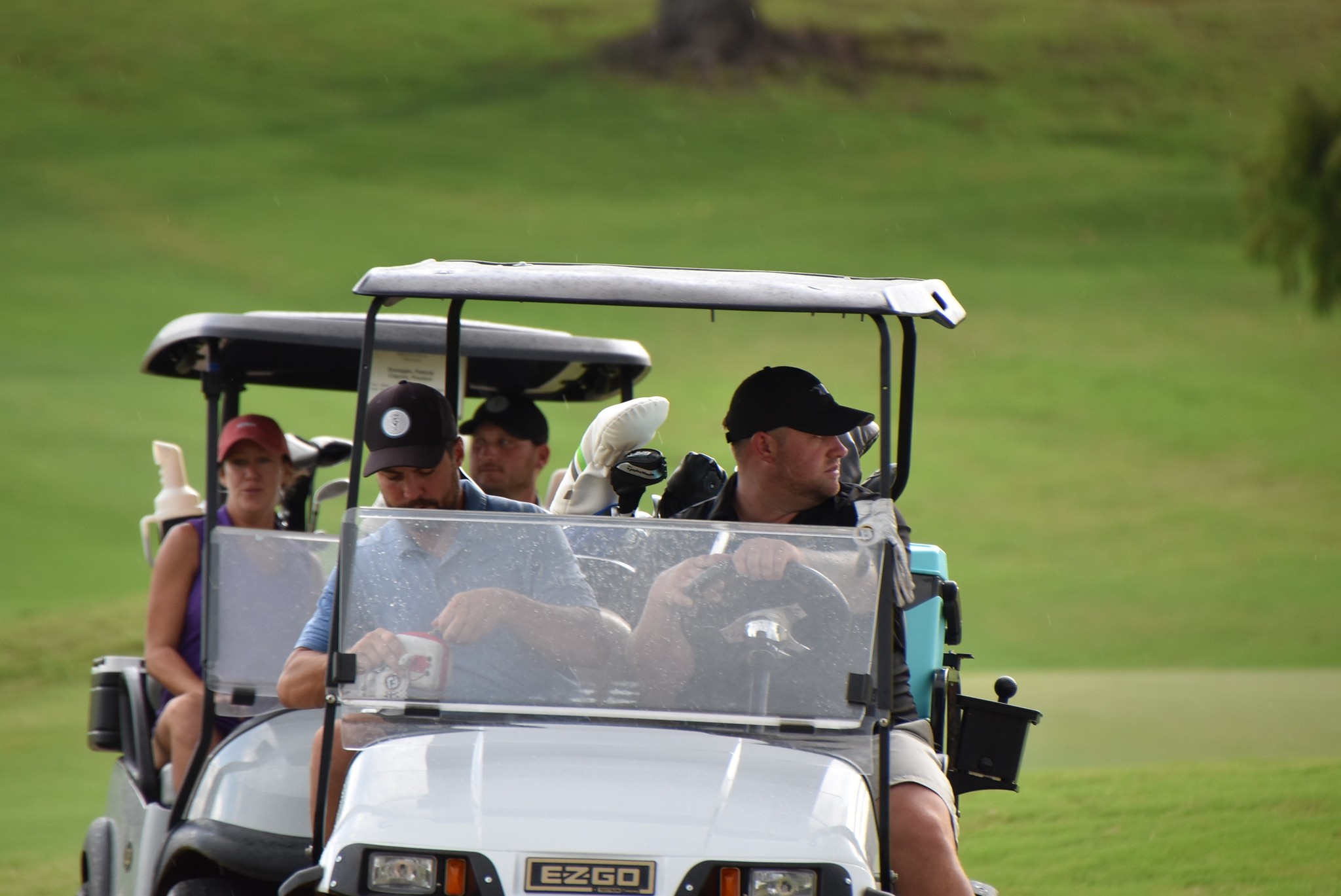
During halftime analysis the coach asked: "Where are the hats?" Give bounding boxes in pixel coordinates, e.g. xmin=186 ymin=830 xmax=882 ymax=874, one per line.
xmin=216 ymin=415 xmax=293 ymax=464
xmin=726 ymin=365 xmax=874 ymax=445
xmin=362 ymin=380 xmax=458 ymax=478
xmin=458 ymin=390 xmax=547 ymax=445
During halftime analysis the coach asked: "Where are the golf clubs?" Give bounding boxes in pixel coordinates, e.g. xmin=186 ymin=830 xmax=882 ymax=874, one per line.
xmin=284 ymin=431 xmax=353 ymax=534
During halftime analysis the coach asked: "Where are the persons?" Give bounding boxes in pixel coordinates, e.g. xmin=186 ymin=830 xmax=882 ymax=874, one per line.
xmin=148 ymin=415 xmax=326 ymax=794
xmin=459 ymin=386 xmax=550 ymax=508
xmin=631 ymin=366 xmax=974 ymax=896
xmin=278 ymin=377 xmax=603 ymax=851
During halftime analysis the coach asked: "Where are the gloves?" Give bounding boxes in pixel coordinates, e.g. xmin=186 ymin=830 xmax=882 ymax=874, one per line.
xmin=851 ymin=497 xmax=917 ymax=607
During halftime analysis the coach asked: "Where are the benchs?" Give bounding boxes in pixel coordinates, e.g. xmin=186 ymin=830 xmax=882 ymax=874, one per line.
xmin=119 ymin=514 xmax=204 ymax=805
